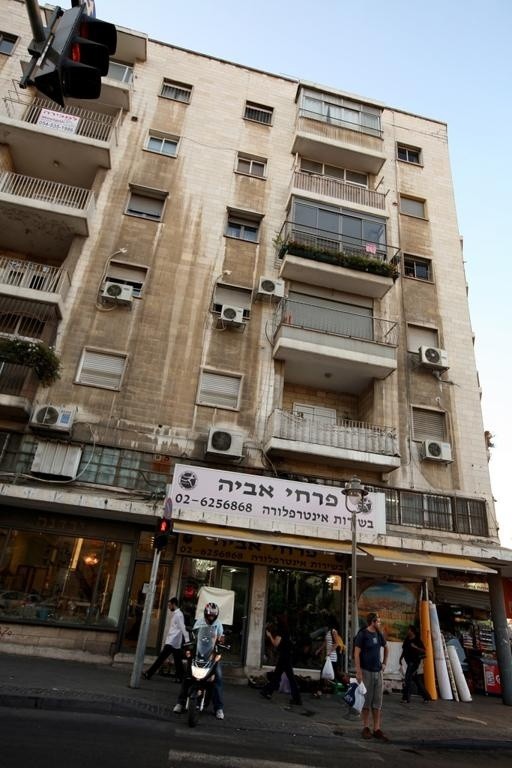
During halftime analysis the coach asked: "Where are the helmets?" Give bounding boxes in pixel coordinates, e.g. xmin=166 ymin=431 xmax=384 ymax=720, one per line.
xmin=204 ymin=603 xmax=219 ymax=625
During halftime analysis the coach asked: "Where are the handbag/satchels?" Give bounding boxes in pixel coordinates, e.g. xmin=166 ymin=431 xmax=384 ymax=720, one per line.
xmin=338 ymin=654 xmax=343 ymax=667
xmin=417 ymin=650 xmax=425 ymax=659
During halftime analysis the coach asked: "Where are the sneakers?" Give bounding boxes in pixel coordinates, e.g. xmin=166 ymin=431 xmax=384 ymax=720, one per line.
xmin=173 ymin=704 xmax=182 ymax=713
xmin=311 ymin=693 xmax=322 ymax=698
xmin=289 ymin=700 xmax=303 ymax=705
xmin=400 ymin=698 xmax=433 ymax=706
xmin=216 ymin=709 xmax=224 ymax=720
xmin=261 ymin=691 xmax=272 ymax=700
xmin=142 ymin=671 xmax=151 ymax=680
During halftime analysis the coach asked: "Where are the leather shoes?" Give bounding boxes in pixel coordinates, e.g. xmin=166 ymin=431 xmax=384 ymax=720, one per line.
xmin=362 ymin=727 xmax=386 ymax=739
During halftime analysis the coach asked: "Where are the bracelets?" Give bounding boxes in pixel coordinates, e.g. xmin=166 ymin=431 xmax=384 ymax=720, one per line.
xmin=382 ymin=663 xmax=386 ymax=665
xmin=319 ymin=646 xmax=323 ymax=651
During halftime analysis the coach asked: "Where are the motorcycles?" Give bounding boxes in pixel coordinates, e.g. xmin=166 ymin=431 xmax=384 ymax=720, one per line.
xmin=183 ymin=628 xmax=228 ymax=728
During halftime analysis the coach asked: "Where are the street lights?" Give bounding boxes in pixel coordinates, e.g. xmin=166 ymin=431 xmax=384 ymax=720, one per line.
xmin=342 ymin=476 xmax=367 ymax=674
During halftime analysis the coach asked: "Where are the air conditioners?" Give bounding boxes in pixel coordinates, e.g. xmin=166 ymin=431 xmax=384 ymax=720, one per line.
xmin=220 ymin=304 xmax=244 ymax=324
xmin=418 ymin=345 xmax=450 ymax=371
xmin=206 ymin=427 xmax=245 ymax=460
xmin=258 ymin=276 xmax=285 ymax=300
xmin=101 ymin=281 xmax=134 ymax=305
xmin=422 ymin=440 xmax=452 ymax=464
xmin=29 ymin=402 xmax=78 ymax=436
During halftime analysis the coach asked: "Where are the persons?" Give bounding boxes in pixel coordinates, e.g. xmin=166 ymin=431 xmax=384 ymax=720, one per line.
xmin=313 ymin=622 xmax=351 ymax=698
xmin=173 ymin=601 xmax=226 ymax=721
xmin=399 ymin=624 xmax=432 ymax=706
xmin=354 ymin=612 xmax=392 ymax=742
xmin=258 ymin=611 xmax=303 ymax=705
xmin=141 ymin=596 xmax=192 ymax=683
